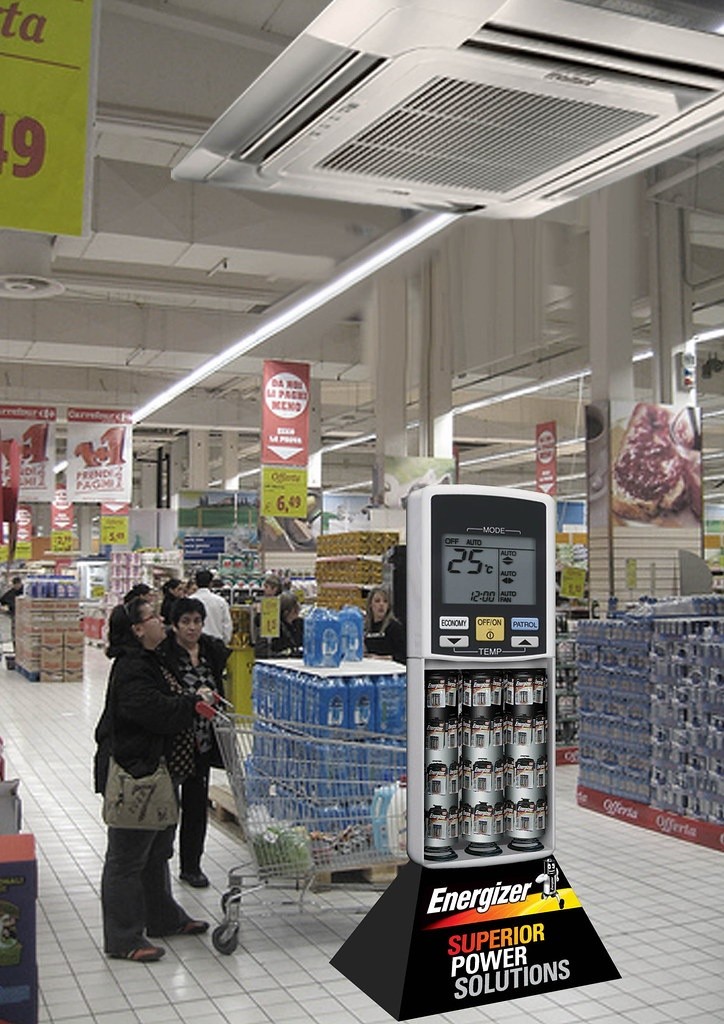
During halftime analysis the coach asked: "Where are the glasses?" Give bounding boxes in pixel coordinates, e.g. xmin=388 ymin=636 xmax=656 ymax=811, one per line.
xmin=143 ymin=611 xmax=158 ymax=621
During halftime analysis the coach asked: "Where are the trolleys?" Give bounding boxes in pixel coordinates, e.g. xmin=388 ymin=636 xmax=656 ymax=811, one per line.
xmin=194 ymin=688 xmax=409 ymax=957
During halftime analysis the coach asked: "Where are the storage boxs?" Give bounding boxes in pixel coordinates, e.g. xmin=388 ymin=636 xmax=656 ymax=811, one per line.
xmin=15 ymin=596 xmax=84 ymax=684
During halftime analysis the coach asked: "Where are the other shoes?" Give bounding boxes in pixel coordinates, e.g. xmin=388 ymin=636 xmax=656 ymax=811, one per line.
xmin=180 ymin=867 xmax=210 ymax=888
xmin=148 ymin=919 xmax=209 ymax=938
xmin=110 ymin=945 xmax=165 ymax=961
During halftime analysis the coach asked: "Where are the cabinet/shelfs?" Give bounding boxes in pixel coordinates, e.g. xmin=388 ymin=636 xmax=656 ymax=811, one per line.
xmin=312 ymin=555 xmax=393 ymax=647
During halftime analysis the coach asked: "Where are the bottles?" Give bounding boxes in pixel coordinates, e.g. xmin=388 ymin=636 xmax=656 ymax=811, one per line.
xmin=241 ymin=607 xmax=408 ymax=834
xmin=370 ymin=774 xmax=409 ymax=859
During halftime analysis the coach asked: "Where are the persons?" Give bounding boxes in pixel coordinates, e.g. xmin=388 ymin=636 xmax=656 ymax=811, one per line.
xmin=0 ymin=577 xmax=23 ymax=651
xmin=121 ymin=570 xmax=233 ymax=706
xmin=254 ymin=576 xmax=304 ymax=658
xmin=365 ymin=590 xmax=406 ymax=666
xmin=154 ymin=597 xmax=227 ymax=888
xmin=93 ymin=599 xmax=210 ymax=960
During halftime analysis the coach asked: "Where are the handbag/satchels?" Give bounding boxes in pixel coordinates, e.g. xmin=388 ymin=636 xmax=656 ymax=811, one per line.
xmin=102 ymin=755 xmax=180 ymax=830
